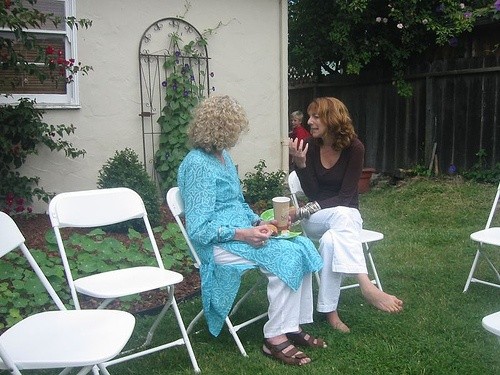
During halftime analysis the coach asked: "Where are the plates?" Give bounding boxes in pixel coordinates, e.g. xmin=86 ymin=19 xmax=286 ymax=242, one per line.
xmin=270 ymin=231 xmax=303 ymax=240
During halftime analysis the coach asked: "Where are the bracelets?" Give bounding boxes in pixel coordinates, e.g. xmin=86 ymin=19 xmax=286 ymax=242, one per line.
xmin=296 ymin=200 xmax=322 ymax=221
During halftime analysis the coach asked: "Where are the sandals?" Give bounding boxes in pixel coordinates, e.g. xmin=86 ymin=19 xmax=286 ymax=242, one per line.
xmin=284 ymin=329 xmax=326 ymax=348
xmin=262 ymin=339 xmax=307 ymax=366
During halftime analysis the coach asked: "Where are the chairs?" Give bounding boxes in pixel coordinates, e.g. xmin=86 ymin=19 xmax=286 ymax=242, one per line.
xmin=288 ymin=171 xmax=383 ymax=293
xmin=462 ymin=184 xmax=500 ymax=292
xmin=0 ymin=211 xmax=135 ymax=375
xmin=166 ymin=187 xmax=268 ymax=357
xmin=48 ymin=187 xmax=201 ymax=374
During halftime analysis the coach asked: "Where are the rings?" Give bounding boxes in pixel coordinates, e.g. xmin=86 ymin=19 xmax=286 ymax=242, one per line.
xmin=261 ymin=241 xmax=264 ymax=246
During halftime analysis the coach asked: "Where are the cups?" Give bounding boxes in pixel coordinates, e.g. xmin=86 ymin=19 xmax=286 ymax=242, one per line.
xmin=272 ymin=197 xmax=291 ymax=227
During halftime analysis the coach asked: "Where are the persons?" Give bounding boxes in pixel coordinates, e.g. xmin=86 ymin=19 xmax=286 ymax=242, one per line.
xmin=289 ymin=111 xmax=311 ymax=170
xmin=176 ymin=94 xmax=328 ymax=367
xmin=287 ymin=96 xmax=404 ymax=334
